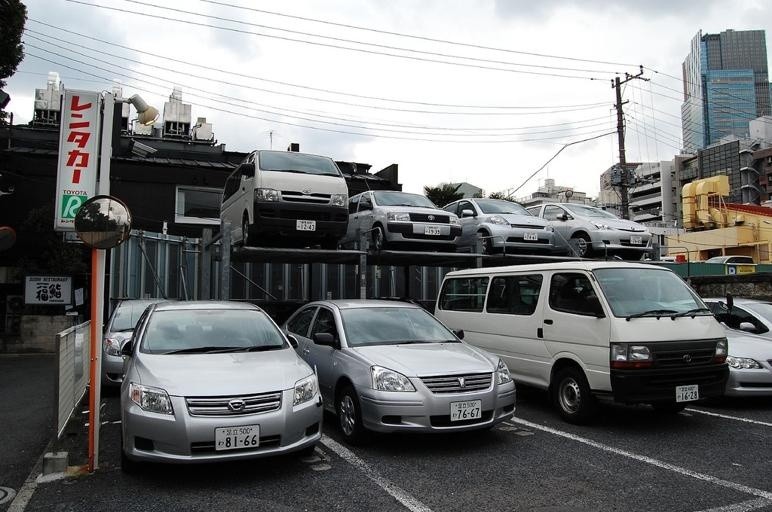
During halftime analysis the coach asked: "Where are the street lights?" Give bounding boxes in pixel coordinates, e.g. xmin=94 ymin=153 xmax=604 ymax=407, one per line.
xmin=92 ymin=94 xmax=161 ymax=470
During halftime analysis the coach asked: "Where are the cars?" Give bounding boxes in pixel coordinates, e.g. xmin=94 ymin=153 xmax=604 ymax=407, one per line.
xmin=528 ymin=202 xmax=651 ymax=261
xmin=344 ymin=191 xmax=464 ymax=254
xmin=120 ymin=302 xmax=322 ymax=474
xmin=102 ymin=297 xmax=175 ymax=384
xmin=281 ymin=296 xmax=517 ymax=446
xmin=678 ymin=296 xmax=772 ymax=396
xmin=445 ymin=197 xmax=554 ymax=255
xmin=705 ymin=255 xmax=753 ymax=263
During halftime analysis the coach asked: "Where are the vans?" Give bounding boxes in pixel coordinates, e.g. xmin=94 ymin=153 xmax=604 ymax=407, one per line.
xmin=220 ymin=149 xmax=350 ymax=251
xmin=433 ymin=261 xmax=731 ymax=425
